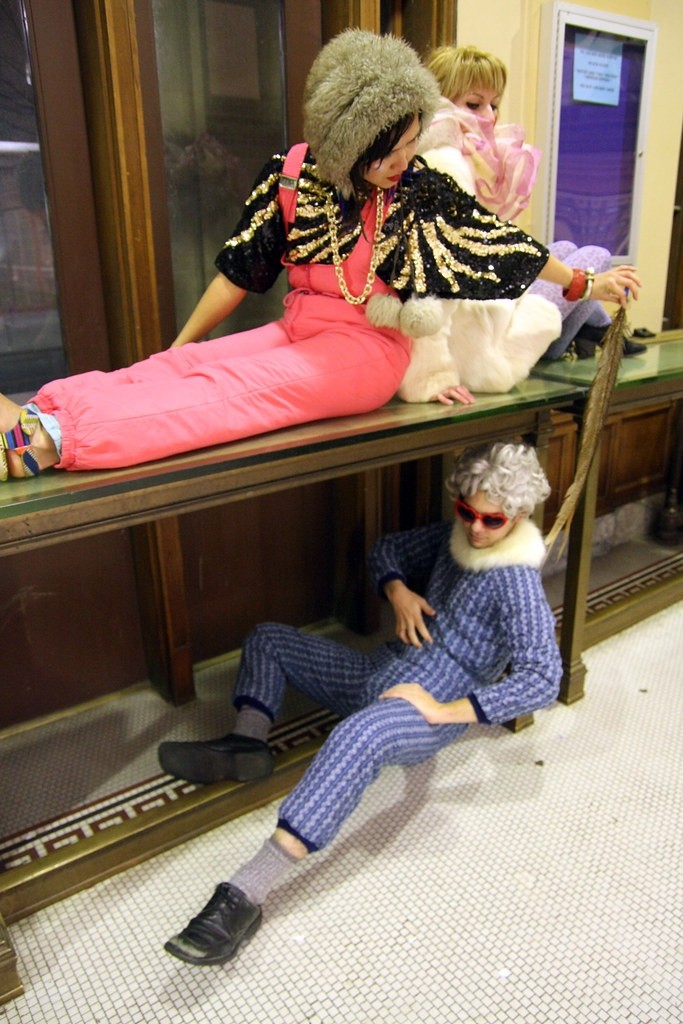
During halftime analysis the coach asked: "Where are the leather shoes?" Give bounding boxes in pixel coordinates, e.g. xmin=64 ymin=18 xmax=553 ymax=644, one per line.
xmin=164 ymin=883 xmax=262 ymax=965
xmin=158 ymin=733 xmax=276 ymax=785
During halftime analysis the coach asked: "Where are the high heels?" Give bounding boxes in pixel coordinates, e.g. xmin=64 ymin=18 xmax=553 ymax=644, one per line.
xmin=574 ymin=320 xmax=647 ymax=358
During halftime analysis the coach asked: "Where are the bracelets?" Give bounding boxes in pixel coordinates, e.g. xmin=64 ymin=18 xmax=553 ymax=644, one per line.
xmin=579 ymin=266 xmax=595 ymax=303
xmin=563 ymin=267 xmax=587 ymax=302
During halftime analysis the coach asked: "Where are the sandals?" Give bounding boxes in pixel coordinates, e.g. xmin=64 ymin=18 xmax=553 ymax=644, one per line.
xmin=0 ymin=393 xmax=40 ymax=481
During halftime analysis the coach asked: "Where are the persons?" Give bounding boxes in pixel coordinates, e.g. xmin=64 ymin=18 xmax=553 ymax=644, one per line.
xmin=1 ymin=28 xmax=642 ymax=481
xmin=158 ymin=442 xmax=562 ymax=968
xmin=398 ymin=44 xmax=647 ymax=407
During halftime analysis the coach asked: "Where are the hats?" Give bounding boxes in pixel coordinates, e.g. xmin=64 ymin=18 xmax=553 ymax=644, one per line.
xmin=304 ymin=30 xmax=437 ymax=192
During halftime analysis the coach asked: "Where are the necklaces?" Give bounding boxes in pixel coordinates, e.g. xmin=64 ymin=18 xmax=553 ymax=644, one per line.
xmin=320 ymin=182 xmax=384 ymax=306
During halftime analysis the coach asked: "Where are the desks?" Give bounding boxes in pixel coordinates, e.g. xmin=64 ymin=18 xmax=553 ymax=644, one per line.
xmin=0 ymin=378 xmax=592 ymax=1006
xmin=533 ymin=328 xmax=683 ymax=708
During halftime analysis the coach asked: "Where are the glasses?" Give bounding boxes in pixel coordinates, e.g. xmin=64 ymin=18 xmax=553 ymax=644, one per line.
xmin=453 ymin=498 xmax=509 ymax=531
